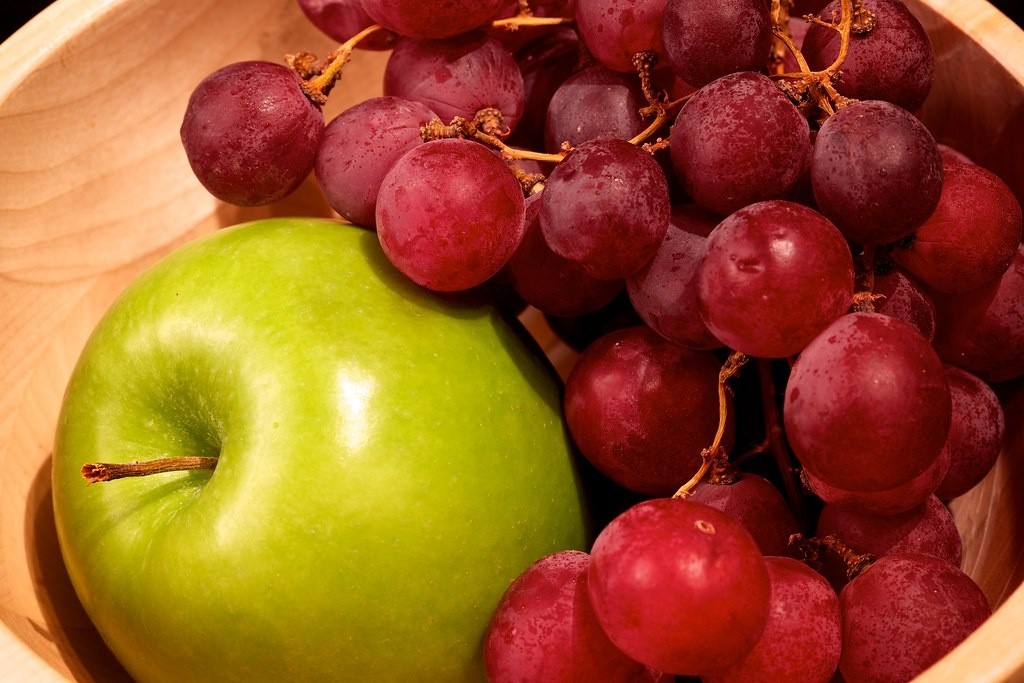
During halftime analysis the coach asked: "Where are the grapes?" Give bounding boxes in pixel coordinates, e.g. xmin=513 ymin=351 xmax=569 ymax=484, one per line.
xmin=177 ymin=0 xmax=1024 ymax=683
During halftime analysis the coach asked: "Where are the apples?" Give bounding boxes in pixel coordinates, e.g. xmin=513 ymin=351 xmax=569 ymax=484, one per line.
xmin=51 ymin=215 xmax=616 ymax=683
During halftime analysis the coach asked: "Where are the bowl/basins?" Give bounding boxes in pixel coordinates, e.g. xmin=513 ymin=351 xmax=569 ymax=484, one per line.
xmin=0 ymin=0 xmax=1024 ymax=683
